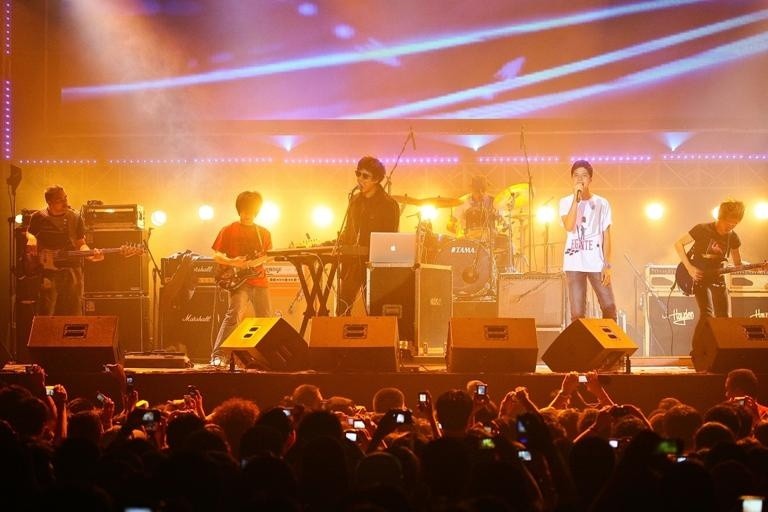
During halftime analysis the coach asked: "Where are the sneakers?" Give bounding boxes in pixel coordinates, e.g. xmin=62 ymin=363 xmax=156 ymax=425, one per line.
xmin=211 ymin=359 xmax=227 ymax=366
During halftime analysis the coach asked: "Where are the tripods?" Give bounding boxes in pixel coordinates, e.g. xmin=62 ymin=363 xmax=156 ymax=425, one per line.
xmin=125 ymin=240 xmax=185 ymax=356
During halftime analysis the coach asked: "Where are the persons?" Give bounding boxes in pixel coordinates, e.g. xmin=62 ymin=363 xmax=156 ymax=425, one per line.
xmin=672 ymin=198 xmax=767 ymax=358
xmin=0 ymin=365 xmax=767 ymax=512
xmin=210 ymin=189 xmax=273 ymax=369
xmin=24 ymin=184 xmax=105 ymax=316
xmin=447 ymin=172 xmax=519 ymax=300
xmin=556 ymin=158 xmax=618 ymax=323
xmin=333 ymin=156 xmax=401 ymax=318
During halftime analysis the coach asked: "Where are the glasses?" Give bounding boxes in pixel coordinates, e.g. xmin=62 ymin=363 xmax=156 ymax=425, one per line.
xmin=355 ymin=170 xmax=374 ymax=179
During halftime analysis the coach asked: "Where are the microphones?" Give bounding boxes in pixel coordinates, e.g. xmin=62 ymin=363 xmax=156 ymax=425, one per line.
xmin=409 ymin=127 xmax=417 ymax=150
xmin=353 ymin=184 xmax=361 ymax=193
xmin=655 ymin=298 xmax=667 ymax=311
xmin=575 ymin=185 xmax=582 ymax=204
xmin=519 ymin=123 xmax=527 ymax=149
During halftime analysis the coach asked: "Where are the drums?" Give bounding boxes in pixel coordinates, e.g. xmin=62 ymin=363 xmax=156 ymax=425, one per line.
xmin=487 ymin=234 xmax=515 ymax=271
xmin=422 ymin=234 xmax=455 ymax=263
xmin=432 ymin=238 xmax=497 ymax=298
xmin=464 ymin=206 xmax=495 ymax=243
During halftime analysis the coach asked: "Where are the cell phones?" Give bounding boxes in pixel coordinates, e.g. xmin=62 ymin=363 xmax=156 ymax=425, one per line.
xmin=95 ymin=392 xmax=107 ymax=402
xmin=737 ymin=496 xmax=766 ymax=511
xmin=347 ymin=417 xmax=354 ymax=425
xmin=518 ymin=450 xmax=532 ymax=461
xmin=126 ymin=376 xmax=134 ymax=394
xmin=479 ymin=437 xmax=496 ymax=449
xmin=392 ymin=412 xmax=410 ymax=424
xmin=516 ymin=420 xmax=529 ymax=434
xmin=608 ymin=438 xmax=623 ymax=449
xmin=476 ymin=384 xmax=488 ymax=401
xmin=141 ymin=410 xmax=161 ymax=423
xmin=344 ymin=432 xmax=362 ymax=442
xmin=353 ymin=420 xmax=367 ymax=429
xmin=45 ymin=385 xmax=56 ymax=396
xmin=576 ymin=374 xmax=590 ymax=384
xmin=734 ymin=396 xmax=751 ymax=407
xmin=282 ymin=408 xmax=294 ymax=417
xmin=676 ymin=455 xmax=688 ymax=463
xmin=417 ymin=391 xmax=428 ymax=407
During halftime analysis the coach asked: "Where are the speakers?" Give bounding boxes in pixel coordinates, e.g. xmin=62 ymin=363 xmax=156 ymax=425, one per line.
xmin=26 ymin=315 xmax=121 ymax=368
xmin=541 ymin=318 xmax=639 ymax=373
xmin=643 ymin=263 xmax=701 ymax=358
xmin=159 ymin=255 xmax=228 ymax=363
xmin=309 ymin=316 xmax=402 ymax=373
xmin=444 ymin=317 xmax=538 ymax=373
xmin=690 ymin=317 xmax=768 ymax=372
xmin=83 ymin=228 xmax=151 ymax=353
xmin=725 ymin=270 xmax=768 ymax=317
xmin=219 ymin=317 xmax=308 ymax=369
xmin=366 ymin=263 xmax=454 ymax=357
xmin=15 ymin=227 xmax=40 ymax=365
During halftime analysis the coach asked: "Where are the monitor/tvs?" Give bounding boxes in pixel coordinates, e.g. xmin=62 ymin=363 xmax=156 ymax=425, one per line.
xmin=369 ymin=231 xmax=416 ymax=267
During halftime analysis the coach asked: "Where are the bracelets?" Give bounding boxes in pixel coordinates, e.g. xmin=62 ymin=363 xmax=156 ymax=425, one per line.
xmin=604 ymin=262 xmax=612 ymax=268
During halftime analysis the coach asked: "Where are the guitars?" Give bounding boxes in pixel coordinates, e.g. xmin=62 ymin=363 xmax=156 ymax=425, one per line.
xmin=675 ymin=256 xmax=768 ymax=295
xmin=23 ymin=240 xmax=144 ymax=277
xmin=215 ymin=233 xmax=322 ymax=292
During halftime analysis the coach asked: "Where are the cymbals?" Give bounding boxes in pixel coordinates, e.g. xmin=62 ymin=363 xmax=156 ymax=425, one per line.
xmin=420 ymin=196 xmax=462 ymax=208
xmin=505 ymin=214 xmax=535 ymax=221
xmin=393 ymin=194 xmax=422 ymax=204
xmin=491 ymin=183 xmax=535 ymax=213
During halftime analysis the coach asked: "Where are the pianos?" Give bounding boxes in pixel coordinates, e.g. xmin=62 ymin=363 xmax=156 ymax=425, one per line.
xmin=266 ymin=244 xmax=369 ymax=263
